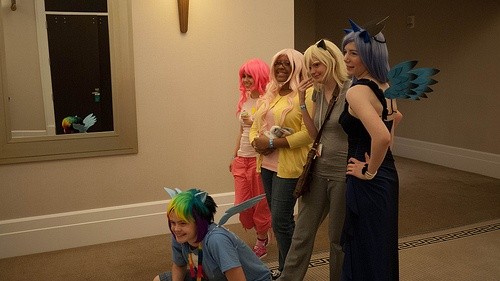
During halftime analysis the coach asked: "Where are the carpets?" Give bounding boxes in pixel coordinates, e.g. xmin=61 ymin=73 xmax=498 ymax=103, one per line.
xmin=151 ymin=217 xmax=500 ymax=281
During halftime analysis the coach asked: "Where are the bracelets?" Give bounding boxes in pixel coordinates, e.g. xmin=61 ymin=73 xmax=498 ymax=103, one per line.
xmin=300 ymin=104 xmax=306 ymax=109
xmin=361 ymin=162 xmax=377 ymax=179
xmin=270 ymin=137 xmax=273 ymax=151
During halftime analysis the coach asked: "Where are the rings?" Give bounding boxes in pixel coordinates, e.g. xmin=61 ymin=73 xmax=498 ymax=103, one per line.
xmin=254 ymin=145 xmax=256 ymax=148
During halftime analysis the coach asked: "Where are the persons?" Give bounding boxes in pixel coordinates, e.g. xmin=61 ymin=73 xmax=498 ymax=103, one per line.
xmin=62 ymin=116 xmax=88 ymax=134
xmin=249 ymin=48 xmax=315 ymax=280
xmin=229 ymin=59 xmax=274 ymax=260
xmin=337 ymin=29 xmax=403 ymax=281
xmin=152 ymin=188 xmax=274 ymax=281
xmin=272 ymin=39 xmax=352 ymax=281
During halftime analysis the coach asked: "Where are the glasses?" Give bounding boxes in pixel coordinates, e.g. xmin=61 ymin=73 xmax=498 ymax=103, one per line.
xmin=274 ymin=60 xmax=290 ymax=68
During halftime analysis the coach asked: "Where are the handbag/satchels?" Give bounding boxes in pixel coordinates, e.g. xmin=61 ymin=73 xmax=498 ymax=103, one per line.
xmin=292 ymin=151 xmax=318 ymax=199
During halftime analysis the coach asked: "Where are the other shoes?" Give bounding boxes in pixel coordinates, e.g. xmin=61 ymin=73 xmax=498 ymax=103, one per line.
xmin=270 ymin=269 xmax=282 ymax=280
xmin=252 ymin=235 xmax=270 ymax=259
